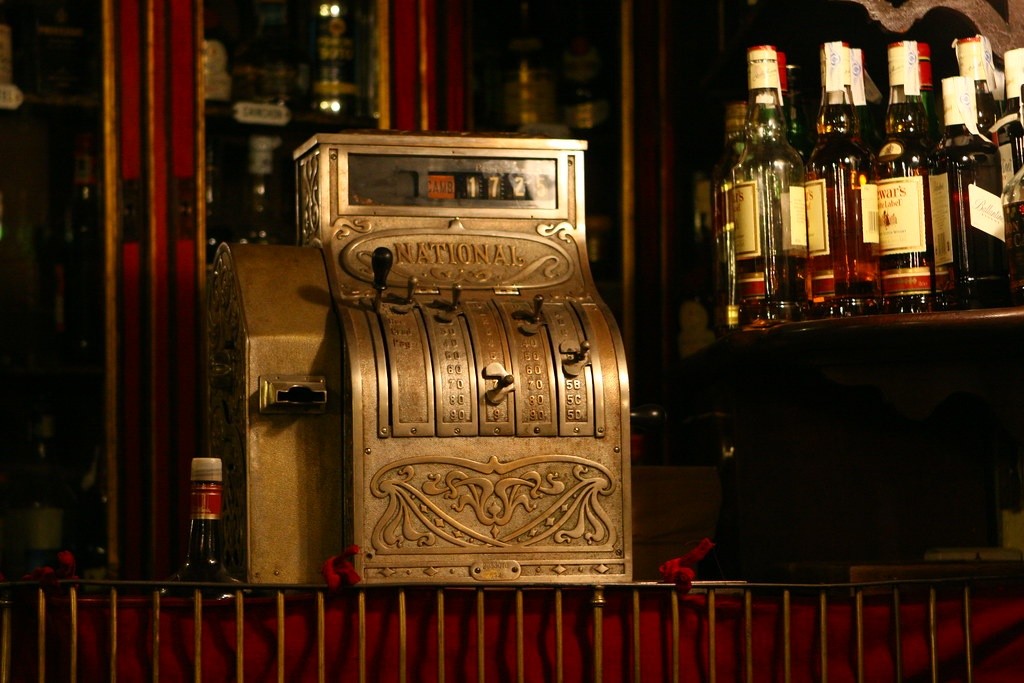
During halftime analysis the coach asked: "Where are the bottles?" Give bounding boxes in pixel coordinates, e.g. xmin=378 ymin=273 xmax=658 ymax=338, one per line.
xmin=0 ymin=0 xmax=106 ymax=579
xmin=678 ymin=34 xmax=1024 ymax=332
xmin=203 ymin=0 xmax=622 ymax=285
xmin=158 ymin=457 xmax=242 ymax=599
